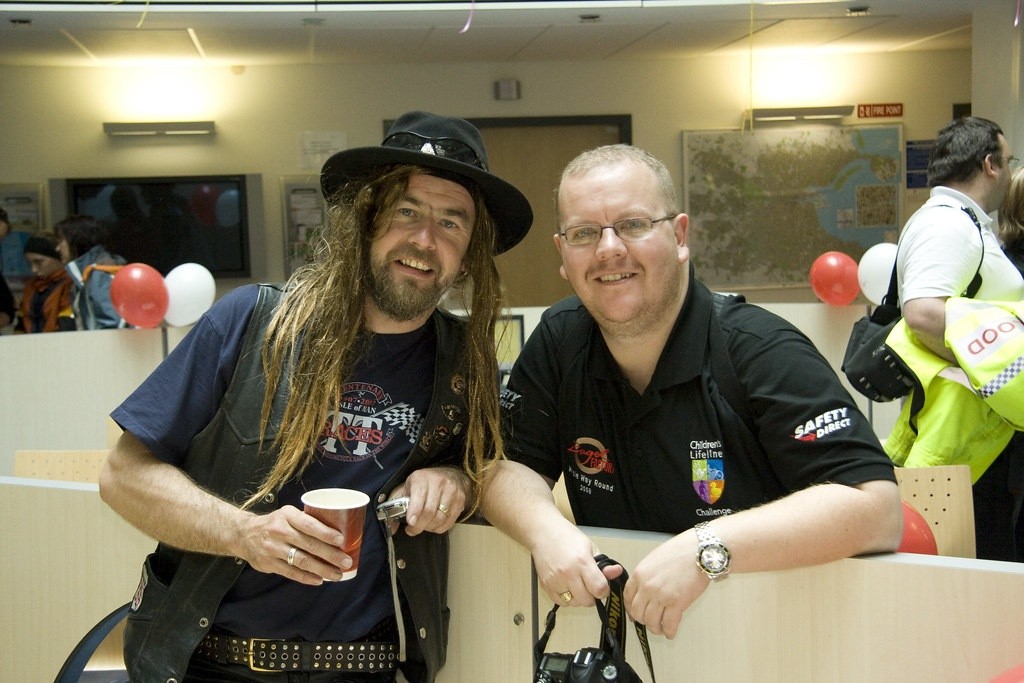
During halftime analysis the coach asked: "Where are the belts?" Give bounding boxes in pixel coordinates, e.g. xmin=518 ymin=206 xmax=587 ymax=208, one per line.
xmin=195 ymin=633 xmax=401 ymax=672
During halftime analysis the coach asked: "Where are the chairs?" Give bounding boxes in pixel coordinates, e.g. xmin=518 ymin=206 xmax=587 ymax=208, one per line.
xmin=890 ymin=464 xmax=976 ymax=561
xmin=18 ymin=449 xmax=129 ymax=671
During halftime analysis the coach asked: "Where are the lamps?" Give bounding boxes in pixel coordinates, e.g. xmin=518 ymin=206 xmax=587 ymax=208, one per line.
xmin=101 ymin=120 xmax=219 ymax=140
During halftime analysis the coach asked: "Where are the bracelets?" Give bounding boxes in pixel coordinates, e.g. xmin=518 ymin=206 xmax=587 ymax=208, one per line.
xmin=91 ymin=262 xmax=98 ymax=268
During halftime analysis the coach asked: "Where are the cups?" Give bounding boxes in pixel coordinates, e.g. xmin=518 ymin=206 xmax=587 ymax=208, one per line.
xmin=301 ymin=487 xmax=369 ymax=582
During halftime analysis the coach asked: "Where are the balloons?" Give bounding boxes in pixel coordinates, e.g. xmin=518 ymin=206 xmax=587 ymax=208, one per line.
xmin=159 ymin=262 xmax=217 ymax=328
xmin=856 ymin=241 xmax=898 ymax=306
xmin=110 ymin=262 xmax=169 ymax=329
xmin=192 ymin=185 xmax=240 ymax=228
xmin=808 ymin=250 xmax=860 ymax=304
xmin=897 ymin=498 xmax=938 ymax=555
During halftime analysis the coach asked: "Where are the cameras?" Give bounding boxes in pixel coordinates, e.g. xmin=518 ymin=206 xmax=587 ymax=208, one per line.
xmin=533 ymin=647 xmax=644 ymax=683
xmin=376 ymin=496 xmax=411 ymax=521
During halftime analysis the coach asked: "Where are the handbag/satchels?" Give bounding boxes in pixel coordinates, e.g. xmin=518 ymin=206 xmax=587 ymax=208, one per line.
xmin=841 ymin=202 xmax=983 ymax=402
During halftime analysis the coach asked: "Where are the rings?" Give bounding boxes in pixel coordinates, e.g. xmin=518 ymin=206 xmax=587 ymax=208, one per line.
xmin=288 ymin=548 xmax=298 ymax=566
xmin=559 ymin=591 xmax=573 ymax=603
xmin=438 ymin=504 xmax=452 ymax=517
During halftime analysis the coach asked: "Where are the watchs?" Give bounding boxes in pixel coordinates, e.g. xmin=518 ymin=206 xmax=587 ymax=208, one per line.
xmin=695 ymin=521 xmax=734 ymax=585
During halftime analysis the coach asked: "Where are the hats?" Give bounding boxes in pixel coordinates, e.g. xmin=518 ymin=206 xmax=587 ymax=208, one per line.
xmin=320 ymin=111 xmax=533 ymax=256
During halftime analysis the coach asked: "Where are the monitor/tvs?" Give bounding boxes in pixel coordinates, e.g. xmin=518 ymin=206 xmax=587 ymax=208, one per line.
xmin=64 ymin=174 xmax=252 ymax=278
xmin=487 ymin=315 xmax=524 ymax=375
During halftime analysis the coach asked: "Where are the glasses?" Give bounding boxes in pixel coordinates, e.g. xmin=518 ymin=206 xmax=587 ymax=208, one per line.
xmin=380 ymin=131 xmax=489 ymax=173
xmin=983 ymin=156 xmax=1020 ymax=168
xmin=559 ymin=214 xmax=677 ymax=245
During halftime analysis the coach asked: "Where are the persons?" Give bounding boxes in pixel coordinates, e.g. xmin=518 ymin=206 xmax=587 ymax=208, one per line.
xmin=0 ymin=205 xmax=131 ymax=336
xmin=899 ymin=116 xmax=1024 ymax=562
xmin=98 ymin=112 xmax=533 ymax=683
xmin=471 ymin=143 xmax=903 ymax=637
